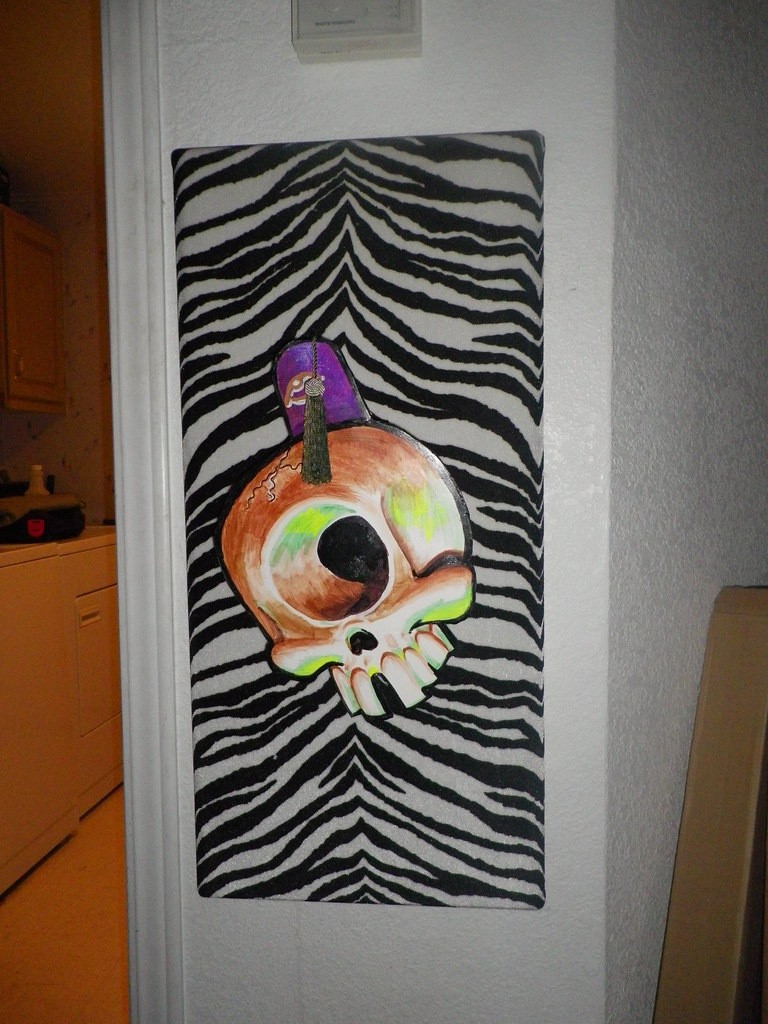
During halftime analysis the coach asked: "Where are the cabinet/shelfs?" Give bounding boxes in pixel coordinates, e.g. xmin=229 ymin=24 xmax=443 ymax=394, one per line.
xmin=0 ymin=205 xmax=66 ymax=415
xmin=1 ymin=543 xmax=119 ymax=893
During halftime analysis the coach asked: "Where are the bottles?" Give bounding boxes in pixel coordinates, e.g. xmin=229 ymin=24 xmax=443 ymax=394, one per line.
xmin=25 ymin=464 xmax=49 ymax=497
